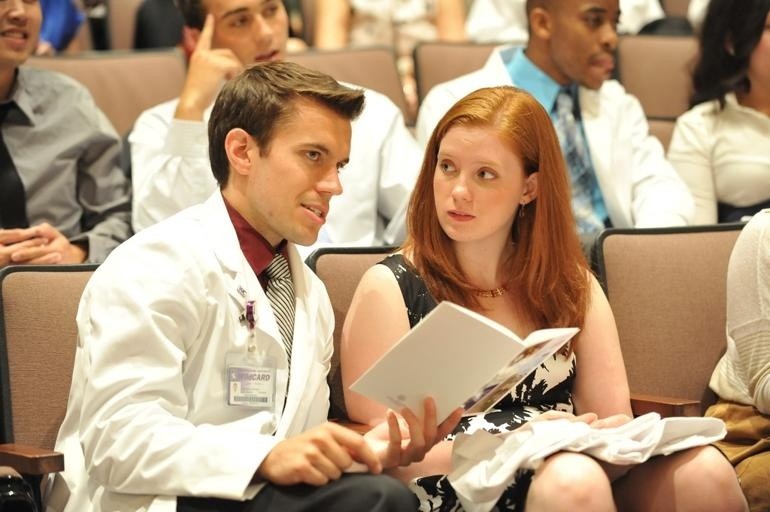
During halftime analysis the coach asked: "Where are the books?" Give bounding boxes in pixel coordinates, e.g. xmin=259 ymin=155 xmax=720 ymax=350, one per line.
xmin=346 ymin=298 xmax=582 ymax=430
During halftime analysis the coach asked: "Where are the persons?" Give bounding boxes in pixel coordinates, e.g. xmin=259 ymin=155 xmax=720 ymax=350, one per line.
xmin=38 ymin=0 xmax=82 ymax=55
xmin=0 ymin=0 xmax=133 ymax=271
xmin=413 ymin=0 xmax=701 ymax=254
xmin=39 ymin=59 xmax=465 ymax=512
xmin=128 ymin=0 xmax=425 ymax=262
xmin=339 ymin=84 xmax=748 ymax=512
xmin=700 ymin=206 xmax=769 ymax=512
xmin=647 ymin=0 xmax=770 ymax=226
xmin=315 ymin=0 xmax=709 ymax=104
xmin=133 ymin=0 xmax=186 ymax=49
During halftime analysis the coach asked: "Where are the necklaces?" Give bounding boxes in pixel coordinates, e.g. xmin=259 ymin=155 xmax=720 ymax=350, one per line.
xmin=449 ymin=272 xmax=541 ymax=301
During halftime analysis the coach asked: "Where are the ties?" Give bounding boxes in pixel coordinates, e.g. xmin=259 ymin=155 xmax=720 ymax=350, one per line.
xmin=557 ymin=91 xmax=606 ymax=266
xmin=0 ymin=102 xmax=31 ymax=228
xmin=266 ymin=254 xmax=295 ymax=398
